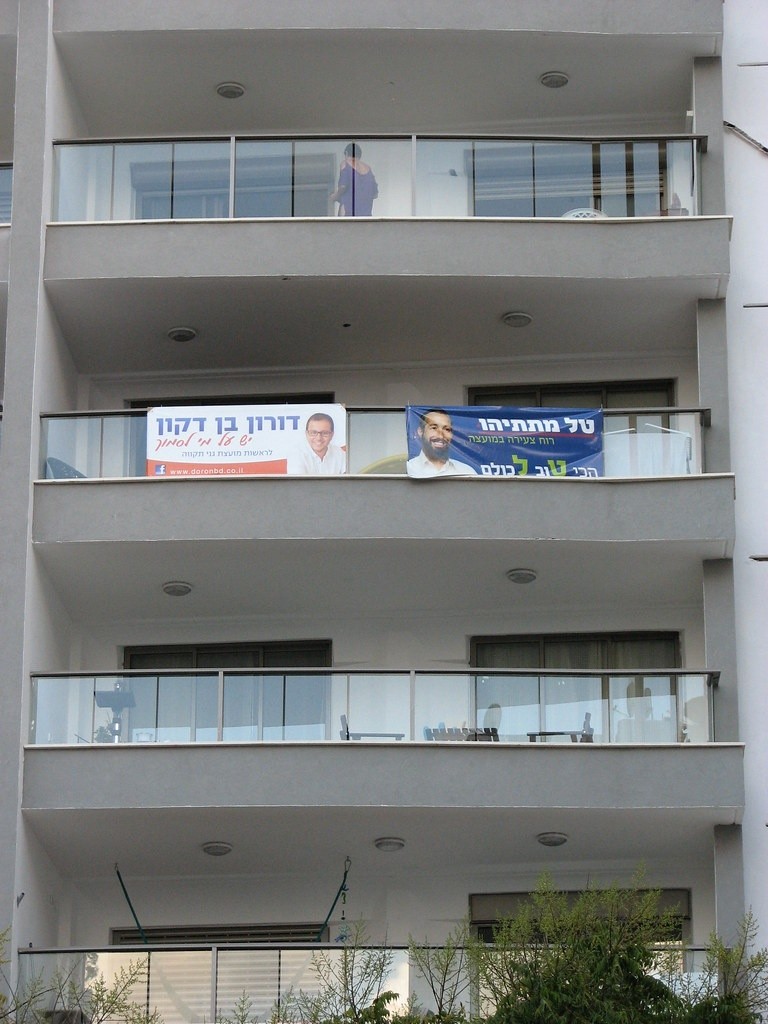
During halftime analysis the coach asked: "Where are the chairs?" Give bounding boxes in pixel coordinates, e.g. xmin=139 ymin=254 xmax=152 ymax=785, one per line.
xmin=340 ymin=714 xmax=405 ymax=742
xmin=528 ymin=712 xmax=594 ymax=742
xmin=563 ymin=206 xmax=608 ymax=219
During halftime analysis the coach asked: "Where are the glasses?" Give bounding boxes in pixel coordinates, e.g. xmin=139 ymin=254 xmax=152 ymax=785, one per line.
xmin=307 ymin=430 xmax=333 ymax=438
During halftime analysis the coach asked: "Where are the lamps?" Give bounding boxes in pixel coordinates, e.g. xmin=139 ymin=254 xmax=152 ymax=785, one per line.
xmin=217 ymin=84 xmax=244 ymax=98
xmin=376 ymin=838 xmax=404 ymax=852
xmin=538 ymin=833 xmax=568 ymax=847
xmin=163 ymin=583 xmax=191 ymax=596
xmin=167 ymin=328 xmax=197 ymax=340
xmin=540 ymin=72 xmax=569 ymax=88
xmin=504 ymin=314 xmax=533 ymax=328
xmin=508 ymin=570 xmax=537 ymax=584
xmin=203 ymin=843 xmax=232 ymax=856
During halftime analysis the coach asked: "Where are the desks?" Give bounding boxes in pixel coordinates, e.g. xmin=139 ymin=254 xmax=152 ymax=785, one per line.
xmin=426 ymin=728 xmax=498 ymax=742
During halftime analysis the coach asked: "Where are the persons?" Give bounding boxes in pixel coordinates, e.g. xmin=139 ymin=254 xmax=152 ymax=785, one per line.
xmin=407 ymin=410 xmax=478 ymax=476
xmin=286 ymin=413 xmax=346 ymax=475
xmin=331 ymin=143 xmax=378 ymax=216
